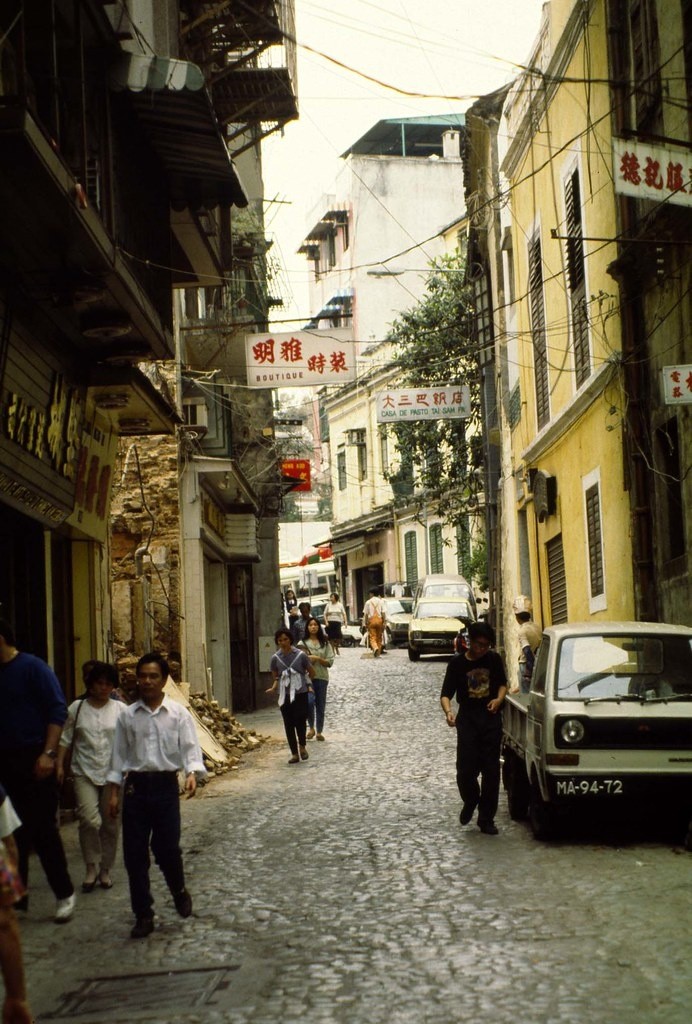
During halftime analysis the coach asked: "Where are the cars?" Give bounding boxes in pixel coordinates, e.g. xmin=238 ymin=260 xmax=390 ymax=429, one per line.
xmin=408 ymin=596 xmax=478 ymax=661
xmin=314 ymin=615 xmax=365 ymax=647
xmin=377 ymin=596 xmax=414 ymax=646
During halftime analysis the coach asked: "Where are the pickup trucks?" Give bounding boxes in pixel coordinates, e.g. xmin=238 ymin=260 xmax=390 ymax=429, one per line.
xmin=497 ymin=621 xmax=692 ymax=840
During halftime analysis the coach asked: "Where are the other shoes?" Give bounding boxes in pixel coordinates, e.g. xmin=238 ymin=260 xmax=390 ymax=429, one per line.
xmin=82 ymin=872 xmax=96 ymax=891
xmin=316 ymin=735 xmax=325 ymax=741
xmin=301 ymin=752 xmax=308 ymax=760
xmin=374 ymin=649 xmax=380 ymax=657
xmin=289 ymin=757 xmax=299 ymax=763
xmin=98 ymin=874 xmax=113 ymax=888
xmin=307 ymin=731 xmax=315 ymax=739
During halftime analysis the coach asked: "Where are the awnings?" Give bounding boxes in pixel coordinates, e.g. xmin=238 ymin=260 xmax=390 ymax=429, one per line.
xmin=304 ymin=287 xmax=353 ymax=330
xmin=313 ymin=518 xmax=394 ymax=548
xmin=296 ymin=199 xmax=349 ymax=254
xmin=108 ymin=49 xmax=248 ymax=211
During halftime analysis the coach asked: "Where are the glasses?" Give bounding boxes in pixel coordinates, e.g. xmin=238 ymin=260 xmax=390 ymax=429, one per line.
xmin=471 ymin=638 xmax=490 ymax=647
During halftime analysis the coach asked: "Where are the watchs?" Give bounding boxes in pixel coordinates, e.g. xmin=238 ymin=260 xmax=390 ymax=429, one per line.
xmin=44 ymin=749 xmax=55 ymax=757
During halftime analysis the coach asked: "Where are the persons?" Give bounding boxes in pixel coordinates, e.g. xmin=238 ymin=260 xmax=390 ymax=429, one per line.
xmin=0 ymin=619 xmax=77 ymax=920
xmin=513 ymin=595 xmax=541 ymax=693
xmin=291 ymin=603 xmax=313 ymax=640
xmin=79 ymin=660 xmax=124 ymax=700
xmin=323 ymin=593 xmax=347 ymax=655
xmin=440 ymin=622 xmax=507 ymax=834
xmin=363 ymin=589 xmax=385 ymax=657
xmin=0 ymin=788 xmax=35 ymax=1024
xmin=106 ymin=655 xmax=196 ymax=938
xmin=284 ymin=590 xmax=300 ymax=627
xmin=297 ymin=618 xmax=334 ymax=742
xmin=265 ymin=628 xmax=314 ymax=763
xmin=55 ymin=664 xmax=127 ymax=891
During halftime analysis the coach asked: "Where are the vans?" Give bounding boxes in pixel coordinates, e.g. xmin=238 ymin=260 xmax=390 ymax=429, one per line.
xmin=411 ymin=574 xmax=478 ymax=621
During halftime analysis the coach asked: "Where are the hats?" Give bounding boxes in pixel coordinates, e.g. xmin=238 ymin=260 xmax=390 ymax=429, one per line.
xmin=513 ymin=594 xmax=532 ymax=618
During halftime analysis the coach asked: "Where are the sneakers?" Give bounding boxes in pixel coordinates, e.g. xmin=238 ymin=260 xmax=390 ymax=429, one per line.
xmin=54 ymin=891 xmax=77 ymax=923
xmin=477 ymin=818 xmax=498 ymax=834
xmin=130 ymin=917 xmax=155 ymax=938
xmin=460 ymin=803 xmax=476 ymax=825
xmin=172 ymin=886 xmax=192 ymax=917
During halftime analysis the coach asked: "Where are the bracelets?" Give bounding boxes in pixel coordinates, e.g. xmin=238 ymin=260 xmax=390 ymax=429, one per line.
xmin=446 ymin=711 xmax=451 ymax=716
xmin=190 ymin=772 xmax=195 ymax=773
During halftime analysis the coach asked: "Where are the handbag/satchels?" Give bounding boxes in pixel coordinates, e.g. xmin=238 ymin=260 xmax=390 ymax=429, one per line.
xmin=63 ymin=744 xmax=75 ymax=781
